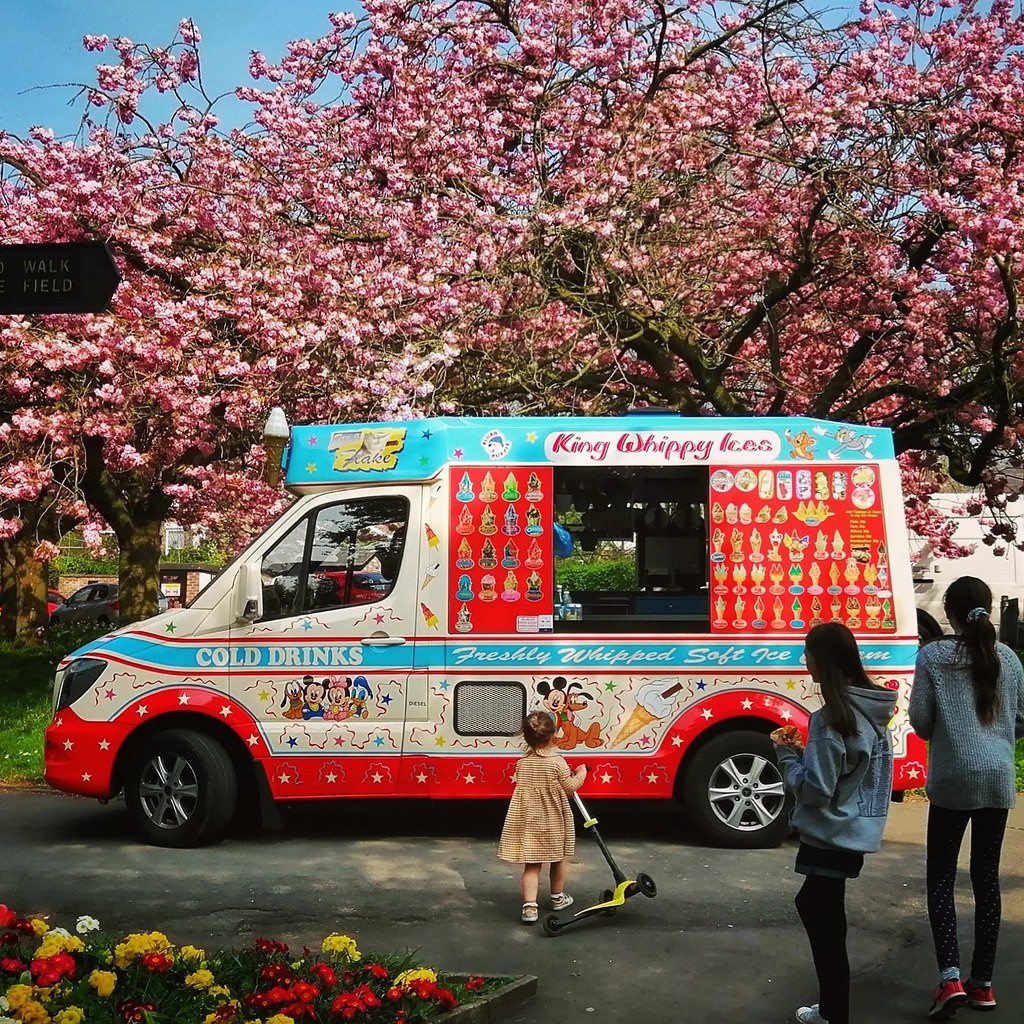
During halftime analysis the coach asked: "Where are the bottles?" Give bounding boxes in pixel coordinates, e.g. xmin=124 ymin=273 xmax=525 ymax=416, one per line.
xmin=553 ymin=585 xmax=564 ymax=621
xmin=565 ymin=603 xmax=583 ymax=620
xmin=562 ymin=587 xmax=572 ymax=621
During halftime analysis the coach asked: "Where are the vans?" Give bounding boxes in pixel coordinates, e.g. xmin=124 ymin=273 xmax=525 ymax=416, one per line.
xmin=903 ymin=492 xmax=1024 ymax=647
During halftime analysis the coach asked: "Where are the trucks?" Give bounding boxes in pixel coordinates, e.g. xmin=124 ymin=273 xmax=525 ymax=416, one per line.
xmin=38 ymin=405 xmax=930 ymax=846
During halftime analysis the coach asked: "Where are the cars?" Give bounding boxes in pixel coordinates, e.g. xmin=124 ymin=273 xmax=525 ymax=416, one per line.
xmin=49 ymin=583 xmax=167 ymax=627
xmin=0 ymin=589 xmax=68 ymax=616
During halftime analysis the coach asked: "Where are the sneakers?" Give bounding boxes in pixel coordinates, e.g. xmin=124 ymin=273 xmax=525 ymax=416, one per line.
xmin=794 ymin=1003 xmax=829 ymax=1024
xmin=928 ymin=979 xmax=966 ymax=1019
xmin=522 ymin=906 xmax=538 ymax=921
xmin=551 ymin=894 xmax=573 ymax=910
xmin=962 ymin=978 xmax=997 ymax=1005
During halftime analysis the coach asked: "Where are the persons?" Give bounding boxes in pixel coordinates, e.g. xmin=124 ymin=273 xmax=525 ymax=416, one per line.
xmin=772 ymin=623 xmax=898 ymax=1024
xmin=908 ymin=576 xmax=1024 ymax=1020
xmin=497 ymin=712 xmax=586 ymax=922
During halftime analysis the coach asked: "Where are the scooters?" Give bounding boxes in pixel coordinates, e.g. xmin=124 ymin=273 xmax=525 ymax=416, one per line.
xmin=543 ymin=766 xmax=659 ymax=935
